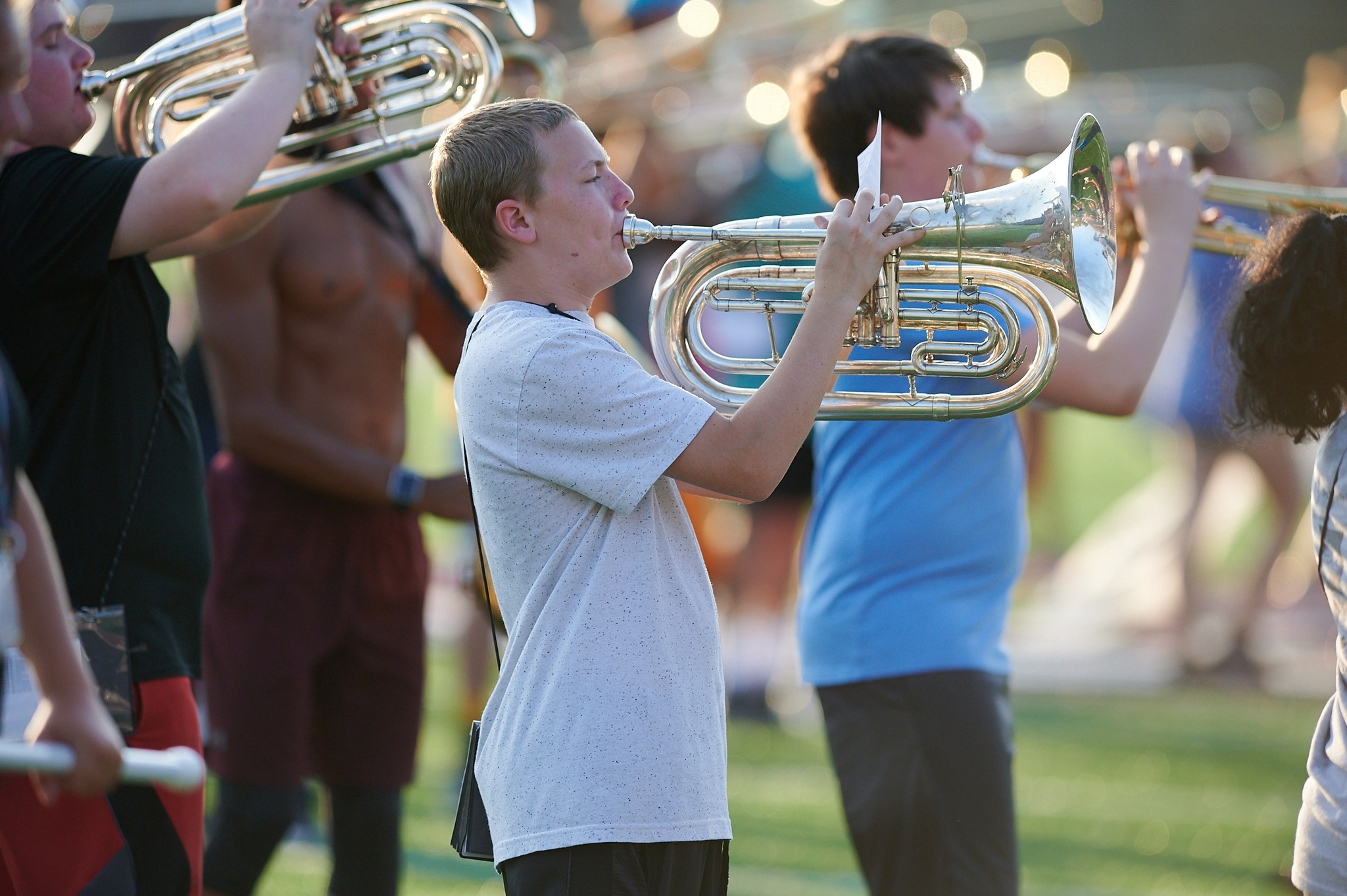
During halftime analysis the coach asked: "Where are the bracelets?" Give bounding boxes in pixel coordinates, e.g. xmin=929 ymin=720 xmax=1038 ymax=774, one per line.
xmin=386 ymin=466 xmax=426 ymax=509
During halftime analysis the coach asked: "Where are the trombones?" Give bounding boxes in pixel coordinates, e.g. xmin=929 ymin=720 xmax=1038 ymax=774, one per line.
xmin=965 ymin=141 xmax=1347 ymax=259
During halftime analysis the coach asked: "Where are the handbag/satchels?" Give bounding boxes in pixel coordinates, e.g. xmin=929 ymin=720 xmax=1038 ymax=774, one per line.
xmin=448 ymin=721 xmax=496 ymax=862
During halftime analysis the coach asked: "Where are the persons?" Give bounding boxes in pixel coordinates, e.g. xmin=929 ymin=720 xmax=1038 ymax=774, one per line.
xmin=1225 ymin=212 xmax=1347 ymax=896
xmin=198 ymin=0 xmax=476 ymax=896
xmin=0 ymin=1 xmax=327 ymax=896
xmin=473 ymin=0 xmax=838 ymax=748
xmin=171 ymin=329 xmax=325 ymax=823
xmin=0 ymin=352 xmax=129 ymax=808
xmin=1175 ymin=127 xmax=1314 ymax=700
xmin=792 ymin=33 xmax=1215 ymax=896
xmin=431 ymin=96 xmax=927 ymax=895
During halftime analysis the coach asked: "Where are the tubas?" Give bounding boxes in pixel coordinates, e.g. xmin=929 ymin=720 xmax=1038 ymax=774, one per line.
xmin=79 ymin=1 xmax=539 ymax=210
xmin=620 ymin=113 xmax=1119 ymax=421
